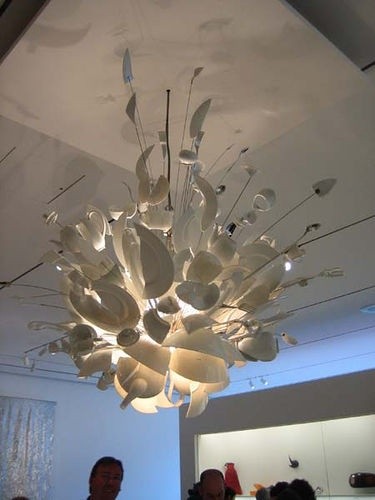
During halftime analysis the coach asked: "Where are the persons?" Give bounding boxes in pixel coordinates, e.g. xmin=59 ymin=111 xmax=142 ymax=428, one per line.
xmin=186 ymin=470 xmax=236 ymax=500
xmin=86 ymin=457 xmax=123 ymax=500
xmin=255 ymin=480 xmax=317 ymax=500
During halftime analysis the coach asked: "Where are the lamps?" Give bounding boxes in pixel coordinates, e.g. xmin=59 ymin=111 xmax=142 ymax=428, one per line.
xmin=19 ymin=48 xmax=344 ymax=420
